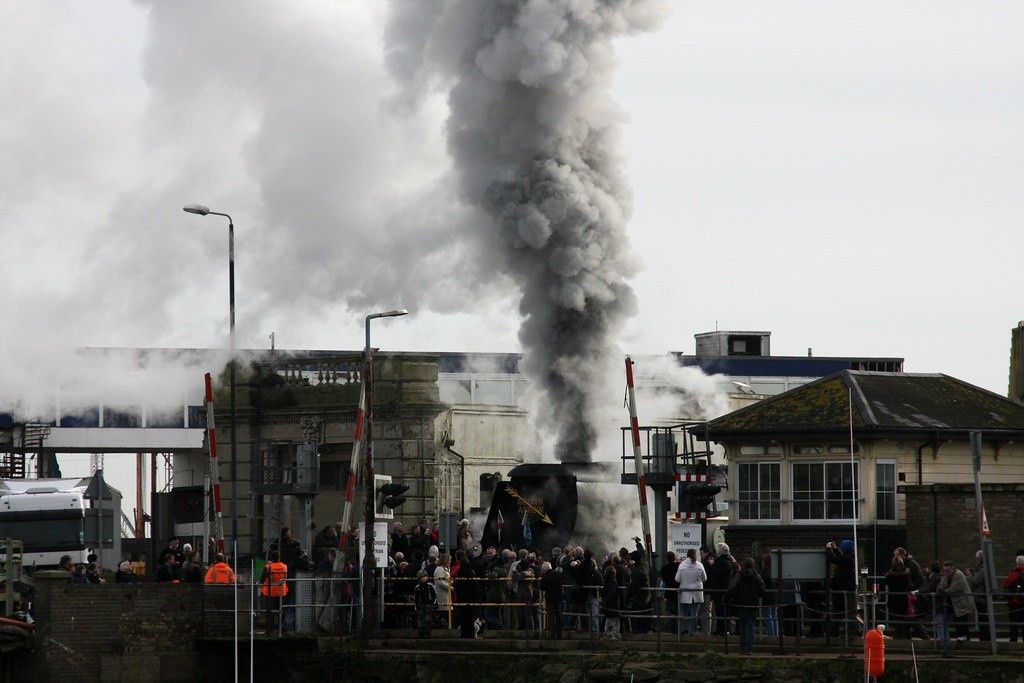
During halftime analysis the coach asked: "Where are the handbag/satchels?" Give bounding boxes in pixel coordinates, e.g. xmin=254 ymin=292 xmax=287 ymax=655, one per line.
xmin=728 ymin=569 xmax=744 ymax=593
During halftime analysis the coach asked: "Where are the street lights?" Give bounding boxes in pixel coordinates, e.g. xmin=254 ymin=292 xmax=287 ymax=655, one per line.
xmin=182 ymin=205 xmax=240 ymax=574
xmin=361 ymin=308 xmax=410 ymax=636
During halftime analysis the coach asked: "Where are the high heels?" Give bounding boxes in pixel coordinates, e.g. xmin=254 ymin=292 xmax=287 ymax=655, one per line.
xmin=681 ymin=630 xmax=689 ymax=635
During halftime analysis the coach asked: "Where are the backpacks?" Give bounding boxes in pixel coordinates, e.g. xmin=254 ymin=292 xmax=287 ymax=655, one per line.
xmin=1008 ymin=571 xmax=1024 ymax=602
xmin=455 ymin=559 xmax=476 ymax=585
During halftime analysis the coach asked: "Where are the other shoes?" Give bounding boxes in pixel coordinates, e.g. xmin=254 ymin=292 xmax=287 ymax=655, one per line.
xmin=416 ymin=632 xmax=423 ymax=638
xmin=746 ymin=650 xmax=752 ymax=655
xmin=423 ymin=632 xmax=430 ymax=638
xmin=957 ymin=636 xmax=967 ymax=642
xmin=517 ymin=627 xmax=526 ymax=630
xmin=603 ymin=637 xmax=611 ymax=641
xmin=488 ymin=625 xmax=496 ymax=630
xmin=557 ymin=637 xmax=562 ymax=640
xmin=739 ymin=650 xmax=744 ymax=655
xmin=609 ymin=637 xmax=619 ymax=642
xmin=497 ymin=624 xmax=504 ymax=630
xmin=547 ymin=637 xmax=555 ymax=640
xmin=578 ymin=627 xmax=589 ymax=633
xmin=562 ymin=624 xmax=569 ymax=630
xmin=568 ymin=625 xmax=576 ymax=629
xmin=369 ymin=633 xmax=384 ymax=639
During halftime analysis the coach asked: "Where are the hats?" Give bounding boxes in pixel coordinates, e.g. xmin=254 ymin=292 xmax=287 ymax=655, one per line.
xmin=840 ymin=540 xmax=854 ymax=552
xmin=416 ymin=570 xmax=428 ymax=578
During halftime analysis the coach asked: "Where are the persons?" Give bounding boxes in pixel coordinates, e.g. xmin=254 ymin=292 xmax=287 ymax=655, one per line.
xmin=57 ymin=518 xmax=1024 ymax=639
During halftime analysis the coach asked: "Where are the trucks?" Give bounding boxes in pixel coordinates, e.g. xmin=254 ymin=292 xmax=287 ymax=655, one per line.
xmin=0 ymin=475 xmax=124 ymax=581
